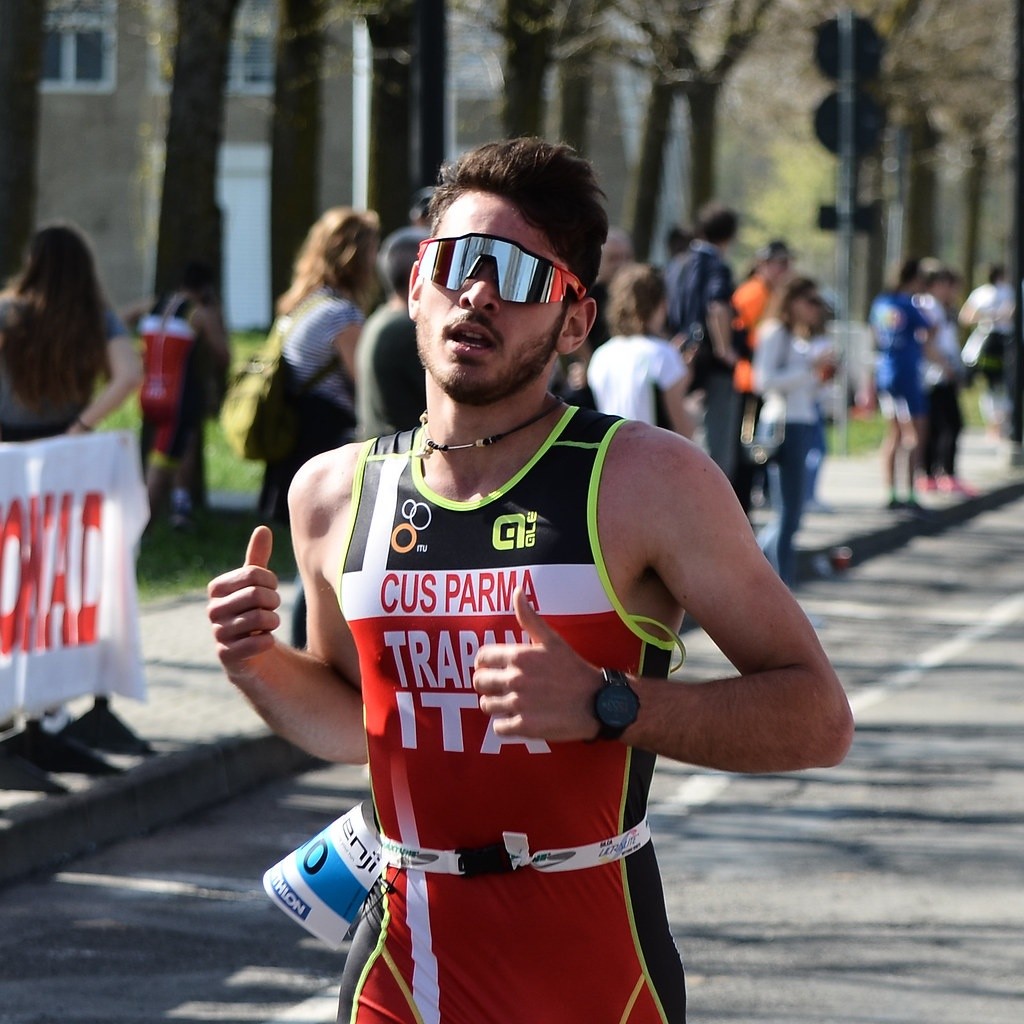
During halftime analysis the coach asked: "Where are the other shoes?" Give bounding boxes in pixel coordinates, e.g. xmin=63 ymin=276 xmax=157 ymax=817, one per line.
xmin=915 ymin=475 xmax=938 ymax=492
xmin=887 ymin=496 xmax=925 ymax=513
xmin=944 ymin=476 xmax=982 ymax=498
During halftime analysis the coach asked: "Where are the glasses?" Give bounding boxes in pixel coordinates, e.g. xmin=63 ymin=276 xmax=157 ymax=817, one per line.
xmin=418 ymin=235 xmax=587 ymax=306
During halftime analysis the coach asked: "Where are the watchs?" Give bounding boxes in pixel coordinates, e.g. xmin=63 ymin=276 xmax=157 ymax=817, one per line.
xmin=583 ymin=668 xmax=640 ymax=746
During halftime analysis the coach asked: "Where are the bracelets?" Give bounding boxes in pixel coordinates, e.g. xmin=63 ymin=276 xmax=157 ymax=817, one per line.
xmin=77 ymin=417 xmax=95 ymax=432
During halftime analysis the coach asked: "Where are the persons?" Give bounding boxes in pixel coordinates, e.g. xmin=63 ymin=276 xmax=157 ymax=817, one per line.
xmin=550 ymin=207 xmax=845 ymax=574
xmin=250 ymin=185 xmax=441 ymax=653
xmin=0 ymin=226 xmax=143 ymax=734
xmin=961 ymin=261 xmax=1016 ymax=436
xmin=869 ymin=258 xmax=976 ymax=511
xmin=208 ymin=136 xmax=853 ymax=1023
xmin=144 ymin=257 xmax=231 ymax=546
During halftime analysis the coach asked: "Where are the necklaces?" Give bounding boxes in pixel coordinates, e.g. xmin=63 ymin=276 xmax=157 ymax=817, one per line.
xmin=418 ymin=396 xmax=564 ymax=458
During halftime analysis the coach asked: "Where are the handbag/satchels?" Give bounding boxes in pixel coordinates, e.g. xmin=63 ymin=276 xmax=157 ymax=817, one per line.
xmin=244 ymin=360 xmax=306 ymax=464
xmin=752 ymin=391 xmax=787 ymax=459
